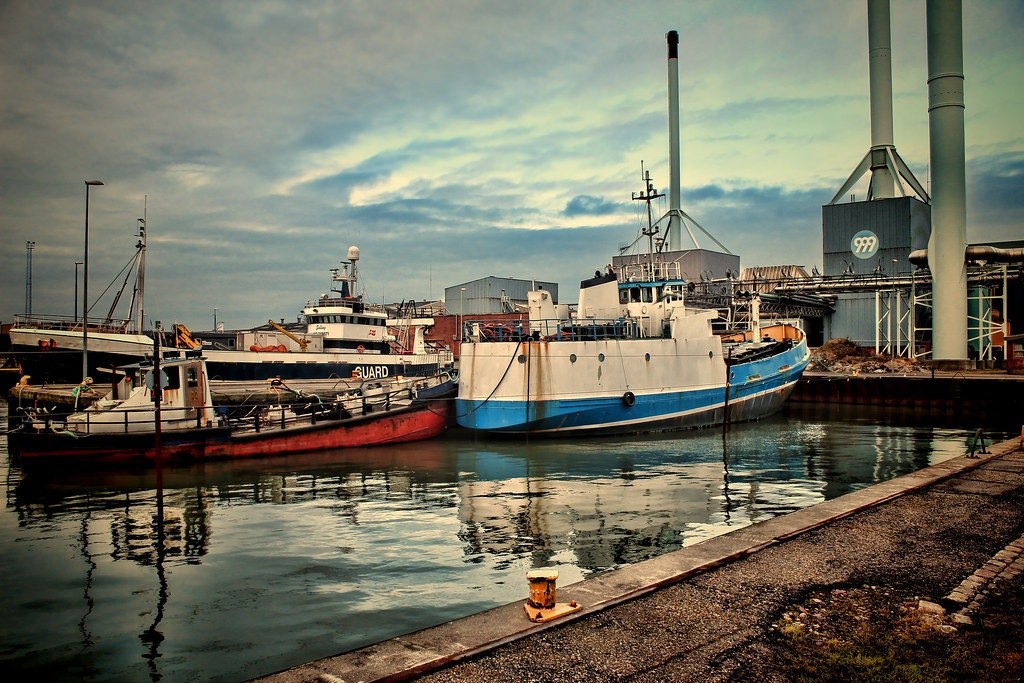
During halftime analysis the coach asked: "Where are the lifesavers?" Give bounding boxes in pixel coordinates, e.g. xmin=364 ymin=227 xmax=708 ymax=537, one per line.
xmin=624 ymin=391 xmax=636 ymax=407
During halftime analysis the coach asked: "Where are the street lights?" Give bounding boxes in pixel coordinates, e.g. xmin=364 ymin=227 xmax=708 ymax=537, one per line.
xmin=214 ymin=308 xmax=219 ymax=331
xmin=83 ymin=180 xmax=105 ymax=380
xmin=75 ymin=262 xmax=83 ymax=322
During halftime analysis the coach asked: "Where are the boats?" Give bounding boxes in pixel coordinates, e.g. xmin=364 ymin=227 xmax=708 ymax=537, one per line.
xmin=9 ymin=240 xmax=155 ymax=356
xmin=8 ymin=247 xmax=459 ymax=468
xmin=454 ymin=159 xmax=812 ymax=439
